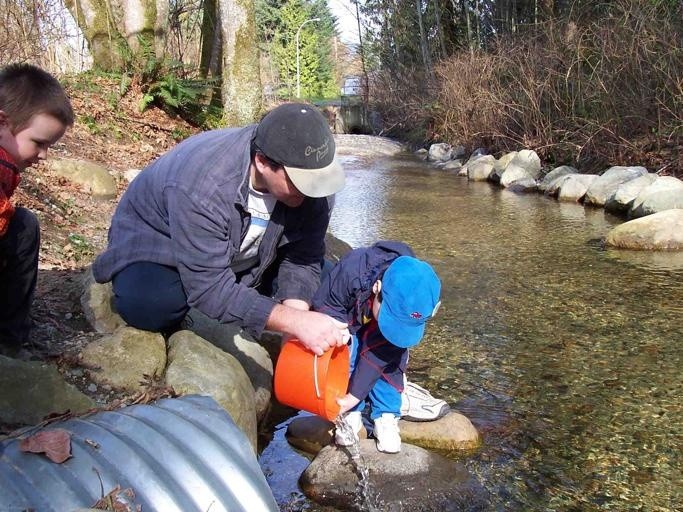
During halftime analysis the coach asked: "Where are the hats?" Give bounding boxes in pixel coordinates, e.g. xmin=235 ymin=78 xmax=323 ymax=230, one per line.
xmin=376 ymin=255 xmax=441 ymax=349
xmin=252 ymin=102 xmax=347 ymax=200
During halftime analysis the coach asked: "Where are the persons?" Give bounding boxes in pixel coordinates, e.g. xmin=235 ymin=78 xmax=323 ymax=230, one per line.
xmin=94 ymin=101 xmax=450 ymax=423
xmin=310 ymin=239 xmax=442 ymax=452
xmin=0 ymin=61 xmax=77 ymax=361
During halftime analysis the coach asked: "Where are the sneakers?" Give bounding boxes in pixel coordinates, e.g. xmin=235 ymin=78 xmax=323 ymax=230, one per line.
xmin=373 ymin=412 xmax=401 ymax=453
xmin=333 ymin=411 xmax=362 ymax=446
xmin=399 ymin=381 xmax=450 ymax=422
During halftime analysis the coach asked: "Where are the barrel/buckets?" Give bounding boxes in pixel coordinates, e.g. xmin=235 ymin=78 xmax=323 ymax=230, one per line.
xmin=273 ymin=335 xmax=355 ymax=421
